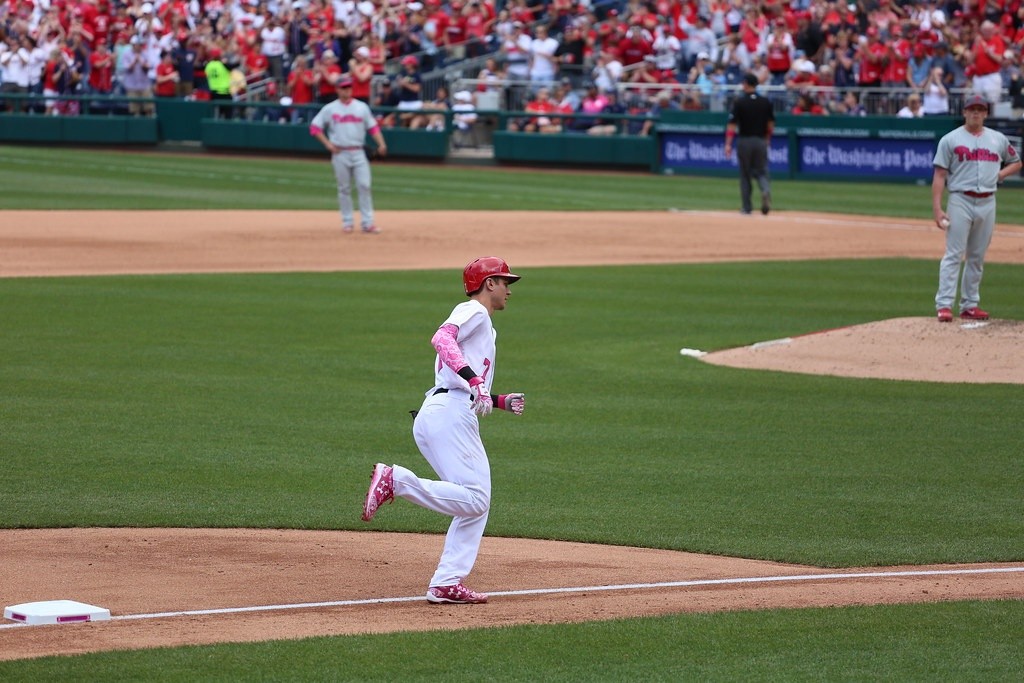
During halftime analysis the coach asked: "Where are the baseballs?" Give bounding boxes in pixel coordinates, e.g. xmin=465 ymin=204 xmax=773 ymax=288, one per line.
xmin=943 ymin=218 xmax=950 ymax=227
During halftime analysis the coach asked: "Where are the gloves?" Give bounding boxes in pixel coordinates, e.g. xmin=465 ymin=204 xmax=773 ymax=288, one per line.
xmin=468 ymin=376 xmax=493 ymax=416
xmin=498 ymin=393 xmax=525 ymax=416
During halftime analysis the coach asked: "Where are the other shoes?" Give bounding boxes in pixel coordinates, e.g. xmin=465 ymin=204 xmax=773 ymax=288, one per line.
xmin=762 ymin=195 xmax=769 ymax=214
xmin=365 ymin=227 xmax=381 ymax=233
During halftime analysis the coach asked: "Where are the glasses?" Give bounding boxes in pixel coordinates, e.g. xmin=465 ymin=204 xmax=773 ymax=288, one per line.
xmin=967 ymin=107 xmax=987 ymax=112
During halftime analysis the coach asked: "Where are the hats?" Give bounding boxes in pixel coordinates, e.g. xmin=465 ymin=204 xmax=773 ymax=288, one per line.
xmin=964 ymin=94 xmax=988 ymax=109
xmin=336 ymin=74 xmax=350 ymax=87
xmin=743 ymin=72 xmax=760 ymax=86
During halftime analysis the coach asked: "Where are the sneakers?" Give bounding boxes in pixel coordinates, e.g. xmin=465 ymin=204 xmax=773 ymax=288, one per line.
xmin=938 ymin=309 xmax=953 ymax=321
xmin=961 ymin=308 xmax=989 ymax=320
xmin=427 ymin=583 xmax=487 ymax=604
xmin=362 ymin=463 xmax=394 ymax=521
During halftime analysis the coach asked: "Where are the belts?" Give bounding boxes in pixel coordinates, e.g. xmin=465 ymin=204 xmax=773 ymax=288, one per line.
xmin=337 ymin=147 xmax=360 ymax=150
xmin=964 ymin=191 xmax=993 ymax=197
xmin=432 ymin=388 xmax=474 ymax=402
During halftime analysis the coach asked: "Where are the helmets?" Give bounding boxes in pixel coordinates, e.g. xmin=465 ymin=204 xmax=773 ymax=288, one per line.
xmin=464 ymin=256 xmax=521 ymax=295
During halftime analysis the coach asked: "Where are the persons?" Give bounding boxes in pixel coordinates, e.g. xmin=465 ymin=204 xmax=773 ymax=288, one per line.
xmin=0 ymin=0 xmax=1024 ymax=140
xmin=724 ymin=74 xmax=775 ymax=215
xmin=930 ymin=94 xmax=1022 ymax=323
xmin=360 ymin=256 xmax=525 ymax=605
xmin=309 ymin=74 xmax=387 ymax=234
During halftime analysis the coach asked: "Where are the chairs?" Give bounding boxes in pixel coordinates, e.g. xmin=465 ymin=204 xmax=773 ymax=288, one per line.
xmin=386 ymin=92 xmax=651 ymax=149
